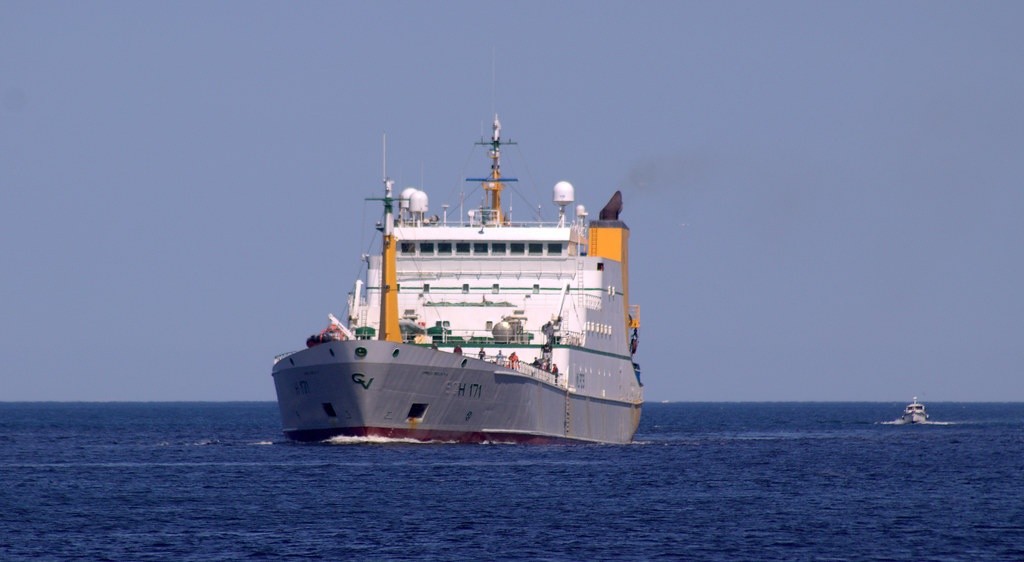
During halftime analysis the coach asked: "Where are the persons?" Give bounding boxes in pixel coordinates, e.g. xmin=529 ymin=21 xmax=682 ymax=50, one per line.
xmin=496 ymin=350 xmax=519 ymax=369
xmin=479 ymin=346 xmax=485 ymax=360
xmin=533 ymin=357 xmax=558 ymax=386
xmin=454 ymin=344 xmax=462 ymax=355
xmin=432 ymin=342 xmax=437 ymax=350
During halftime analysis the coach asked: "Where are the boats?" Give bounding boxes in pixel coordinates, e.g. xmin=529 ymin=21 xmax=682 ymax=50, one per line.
xmin=901 ymin=396 xmax=930 ymax=424
xmin=268 ymin=111 xmax=645 ymax=450
